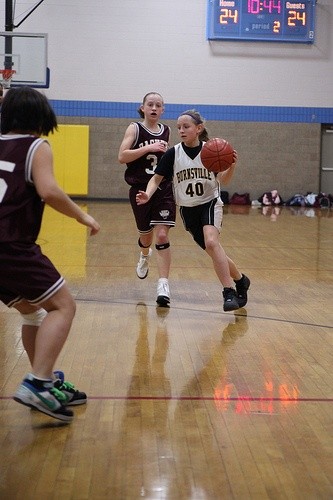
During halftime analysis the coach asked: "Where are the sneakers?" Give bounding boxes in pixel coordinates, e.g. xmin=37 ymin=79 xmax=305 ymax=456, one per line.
xmin=136 ymin=247 xmax=152 ymax=279
xmin=222 ymin=287 xmax=239 ymax=311
xmin=234 ymin=273 xmax=250 ymax=307
xmin=156 ymin=278 xmax=170 ymax=304
xmin=13 ymin=373 xmax=73 ymax=421
xmin=53 ymin=371 xmax=87 ymax=405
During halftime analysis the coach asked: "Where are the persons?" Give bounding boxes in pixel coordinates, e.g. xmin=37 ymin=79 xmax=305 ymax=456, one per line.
xmin=118 ymin=91 xmax=177 ymax=309
xmin=0 ymin=85 xmax=100 ymax=421
xmin=135 ymin=109 xmax=251 ymax=312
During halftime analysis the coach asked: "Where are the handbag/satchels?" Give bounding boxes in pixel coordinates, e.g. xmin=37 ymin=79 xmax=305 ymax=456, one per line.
xmin=230 ymin=192 xmax=250 ymax=205
xmin=258 ymin=190 xmax=283 ymax=205
xmin=286 ymin=192 xmax=333 ymax=207
xmin=221 ymin=191 xmax=229 ymax=204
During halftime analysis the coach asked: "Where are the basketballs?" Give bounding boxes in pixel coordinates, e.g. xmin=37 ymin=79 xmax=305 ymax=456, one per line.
xmin=199 ymin=137 xmax=235 ymax=173
